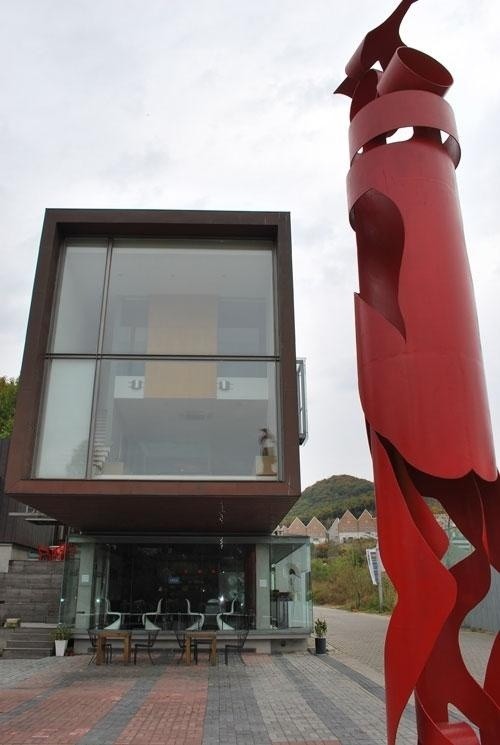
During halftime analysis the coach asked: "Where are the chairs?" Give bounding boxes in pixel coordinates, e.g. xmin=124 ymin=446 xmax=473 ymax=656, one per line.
xmin=88 ymin=621 xmax=252 ymax=666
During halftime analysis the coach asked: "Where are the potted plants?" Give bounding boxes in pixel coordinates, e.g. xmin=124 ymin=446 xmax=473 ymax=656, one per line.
xmin=314 ymin=619 xmax=327 ymax=654
xmin=48 ymin=622 xmax=74 ymax=656
